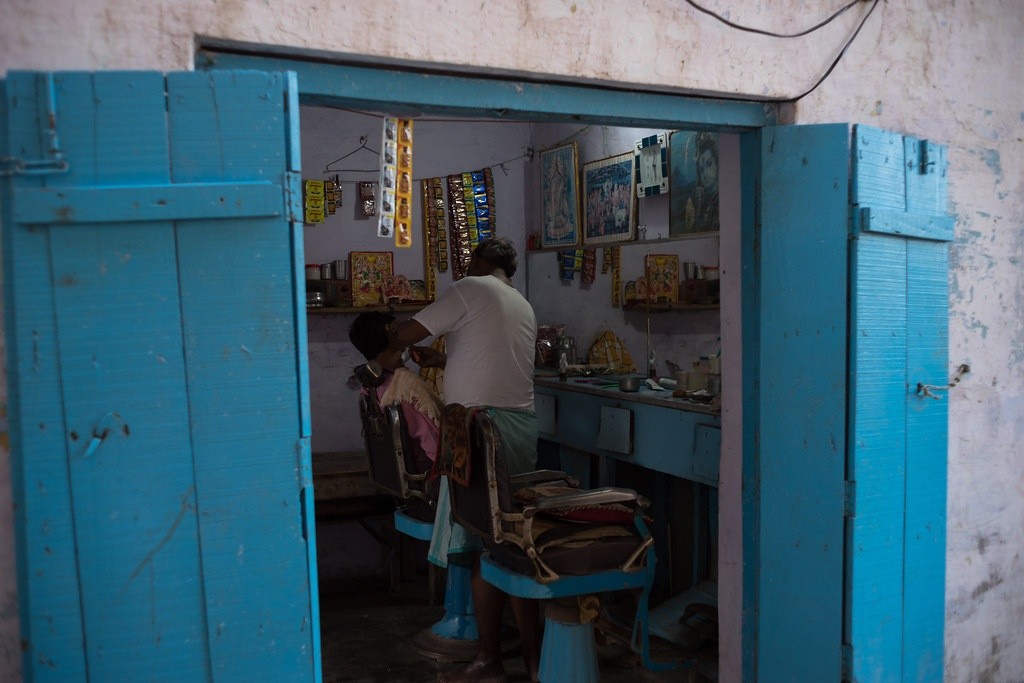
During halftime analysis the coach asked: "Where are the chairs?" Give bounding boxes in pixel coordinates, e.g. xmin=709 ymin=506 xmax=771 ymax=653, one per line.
xmin=446 ymin=404 xmax=697 ymax=683
xmin=359 ymin=394 xmax=437 ymax=608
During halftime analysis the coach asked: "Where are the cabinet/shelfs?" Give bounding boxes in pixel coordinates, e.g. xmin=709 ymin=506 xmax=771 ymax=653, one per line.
xmin=306 ymin=280 xmax=431 ymax=313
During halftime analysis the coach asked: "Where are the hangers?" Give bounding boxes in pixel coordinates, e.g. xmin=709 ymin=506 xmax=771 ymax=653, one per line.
xmin=322 ymin=135 xmax=380 ymax=173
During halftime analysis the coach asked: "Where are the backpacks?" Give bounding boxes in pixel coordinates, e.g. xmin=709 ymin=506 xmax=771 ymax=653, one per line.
xmin=588 ymin=330 xmax=636 ymax=375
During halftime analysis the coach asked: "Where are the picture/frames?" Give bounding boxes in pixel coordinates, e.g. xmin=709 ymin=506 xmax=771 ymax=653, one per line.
xmin=581 ymin=150 xmax=639 ymax=245
xmin=668 ymin=130 xmax=719 ymax=238
xmin=540 ymin=141 xmax=583 ymax=250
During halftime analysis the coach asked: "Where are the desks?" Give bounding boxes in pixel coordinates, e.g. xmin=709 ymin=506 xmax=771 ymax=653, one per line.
xmin=532 ymin=366 xmax=722 ymax=594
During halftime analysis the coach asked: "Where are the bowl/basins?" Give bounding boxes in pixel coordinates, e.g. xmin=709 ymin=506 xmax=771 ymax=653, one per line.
xmin=619 ymin=376 xmax=640 ymax=392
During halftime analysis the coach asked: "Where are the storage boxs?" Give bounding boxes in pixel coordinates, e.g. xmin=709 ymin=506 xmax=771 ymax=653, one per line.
xmin=347 ymin=251 xmax=394 ymax=307
xmin=645 ymin=254 xmax=679 ymax=303
xmin=679 ymin=280 xmax=707 ymax=305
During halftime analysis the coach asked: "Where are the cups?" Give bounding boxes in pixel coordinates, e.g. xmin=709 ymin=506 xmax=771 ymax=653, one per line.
xmin=684 ymin=262 xmax=695 ymax=280
xmin=333 ymin=261 xmax=347 ymax=280
xmin=695 ymin=265 xmax=705 ymax=280
xmin=676 ymin=371 xmax=689 ymax=389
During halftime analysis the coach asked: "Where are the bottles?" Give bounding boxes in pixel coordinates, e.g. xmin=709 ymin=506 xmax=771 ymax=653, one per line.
xmin=689 ymin=361 xmax=704 ymax=390
xmin=559 ymin=354 xmax=568 ymax=381
